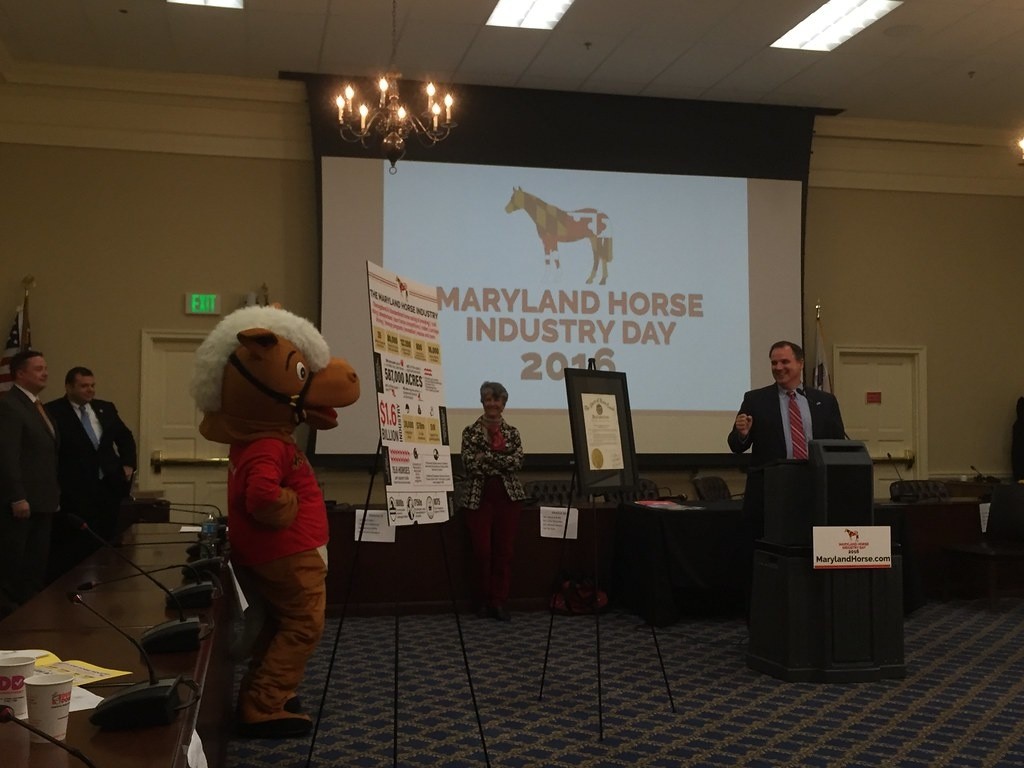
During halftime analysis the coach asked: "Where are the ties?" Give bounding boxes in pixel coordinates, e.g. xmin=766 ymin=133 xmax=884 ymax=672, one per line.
xmin=34 ymin=398 xmax=55 ymax=438
xmin=786 ymin=391 xmax=807 ymax=459
xmin=79 ymin=405 xmax=98 ymax=450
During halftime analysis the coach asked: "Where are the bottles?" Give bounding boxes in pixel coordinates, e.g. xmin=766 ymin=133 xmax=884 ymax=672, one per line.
xmin=200 ymin=514 xmax=217 ymax=559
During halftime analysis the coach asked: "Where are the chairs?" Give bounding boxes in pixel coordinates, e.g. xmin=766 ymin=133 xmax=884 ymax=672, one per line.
xmin=692 ymin=475 xmax=732 ymax=501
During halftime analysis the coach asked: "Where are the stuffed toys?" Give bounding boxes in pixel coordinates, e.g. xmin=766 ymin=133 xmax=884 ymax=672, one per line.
xmin=186 ymin=301 xmax=360 ymax=740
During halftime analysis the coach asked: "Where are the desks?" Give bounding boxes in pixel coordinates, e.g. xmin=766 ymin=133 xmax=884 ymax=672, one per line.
xmin=872 ymin=496 xmax=1001 ymax=615
xmin=0 ymin=522 xmax=230 ymax=768
xmin=325 ymin=497 xmax=619 ymax=620
xmin=616 ymin=498 xmax=924 ymax=617
xmin=945 ymin=480 xmax=1001 ymax=498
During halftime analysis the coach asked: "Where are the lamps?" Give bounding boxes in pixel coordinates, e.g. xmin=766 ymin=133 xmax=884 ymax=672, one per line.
xmin=334 ymin=0 xmax=459 ymax=175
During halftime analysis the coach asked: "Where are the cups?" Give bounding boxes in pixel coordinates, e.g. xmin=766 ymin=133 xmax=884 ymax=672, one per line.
xmin=22 ymin=674 xmax=76 ymax=744
xmin=1 ymin=656 xmax=37 ymax=716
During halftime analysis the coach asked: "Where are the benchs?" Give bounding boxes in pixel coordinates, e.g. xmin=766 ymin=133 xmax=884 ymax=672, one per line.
xmin=524 ymin=479 xmax=661 ymax=503
xmin=889 ymin=478 xmax=953 ymax=503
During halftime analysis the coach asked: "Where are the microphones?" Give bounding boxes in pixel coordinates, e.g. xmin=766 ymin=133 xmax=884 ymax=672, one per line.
xmin=886 ymin=453 xmax=916 ymax=503
xmin=796 ymin=388 xmax=851 ymax=440
xmin=970 ymin=466 xmax=985 ymax=479
xmin=0 ymin=498 xmax=227 ymax=768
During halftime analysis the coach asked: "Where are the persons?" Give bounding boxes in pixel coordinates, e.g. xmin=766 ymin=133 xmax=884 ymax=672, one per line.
xmin=461 ymin=382 xmax=528 ymax=621
xmin=42 ymin=366 xmax=137 ymax=569
xmin=727 ymin=341 xmax=846 ymax=649
xmin=1011 ymin=396 xmax=1024 ymax=486
xmin=0 ymin=350 xmax=60 ymax=623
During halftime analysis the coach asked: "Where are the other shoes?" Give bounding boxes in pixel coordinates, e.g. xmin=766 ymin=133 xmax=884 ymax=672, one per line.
xmin=477 ymin=601 xmax=490 ymax=619
xmin=491 ymin=607 xmax=511 ymax=622
